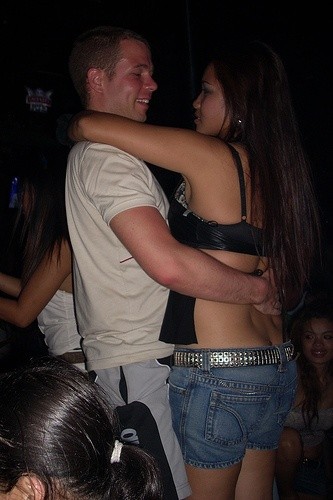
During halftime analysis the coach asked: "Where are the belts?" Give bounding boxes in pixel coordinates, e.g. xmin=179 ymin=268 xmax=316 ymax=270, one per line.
xmin=59 ymin=352 xmax=86 ymax=364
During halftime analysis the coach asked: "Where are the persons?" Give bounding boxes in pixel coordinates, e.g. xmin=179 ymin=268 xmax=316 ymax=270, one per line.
xmin=54 ymin=38 xmax=324 ymax=500
xmin=0 ymin=349 xmax=165 ymax=500
xmin=64 ymin=27 xmax=283 ymax=500
xmin=274 ymin=302 xmax=333 ymax=500
xmin=0 ymin=170 xmax=88 ymax=374
